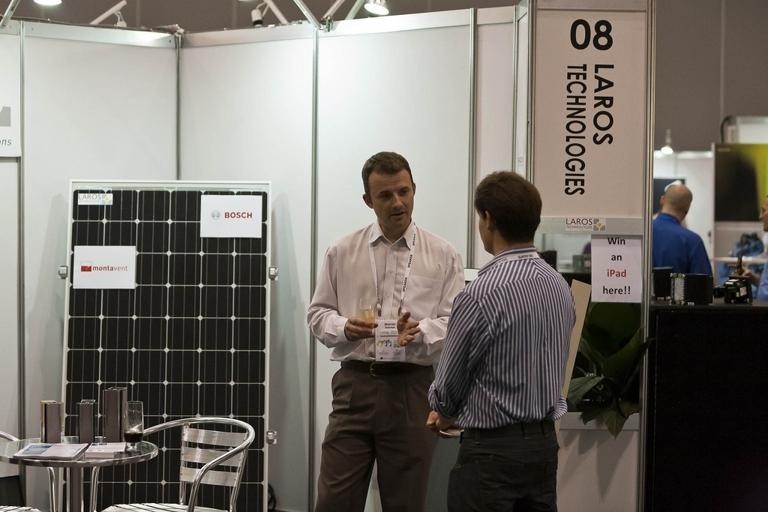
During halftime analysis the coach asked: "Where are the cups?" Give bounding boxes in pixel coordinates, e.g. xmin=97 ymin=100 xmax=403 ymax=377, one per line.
xmin=124 ymin=400 xmax=144 ymax=452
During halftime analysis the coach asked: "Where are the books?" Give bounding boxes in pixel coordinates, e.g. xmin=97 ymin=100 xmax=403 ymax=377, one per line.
xmin=13 ymin=442 xmax=89 ymax=459
xmin=84 ymin=442 xmax=126 ymax=458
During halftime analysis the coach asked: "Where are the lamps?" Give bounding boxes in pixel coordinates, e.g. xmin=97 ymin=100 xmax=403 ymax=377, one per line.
xmin=363 ymin=0 xmax=390 ymax=16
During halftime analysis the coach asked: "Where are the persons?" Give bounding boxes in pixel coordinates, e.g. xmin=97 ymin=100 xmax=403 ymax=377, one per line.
xmin=305 ymin=150 xmax=466 ymax=512
xmin=652 ymin=184 xmax=713 ymax=274
xmin=730 ymin=194 xmax=768 ymax=302
xmin=424 ymin=170 xmax=578 ymax=511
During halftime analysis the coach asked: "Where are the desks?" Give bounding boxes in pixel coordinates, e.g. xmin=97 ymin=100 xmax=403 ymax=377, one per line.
xmin=0 ymin=436 xmax=159 ymax=512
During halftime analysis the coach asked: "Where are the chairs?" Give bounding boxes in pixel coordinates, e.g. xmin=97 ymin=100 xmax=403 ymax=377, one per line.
xmin=89 ymin=418 xmax=255 ymax=512
xmin=0 ymin=429 xmax=55 ymax=511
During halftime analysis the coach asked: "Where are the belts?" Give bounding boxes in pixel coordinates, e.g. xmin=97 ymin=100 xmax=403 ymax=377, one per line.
xmin=340 ymin=359 xmax=434 ymax=379
xmin=461 ymin=423 xmax=555 ymax=439
